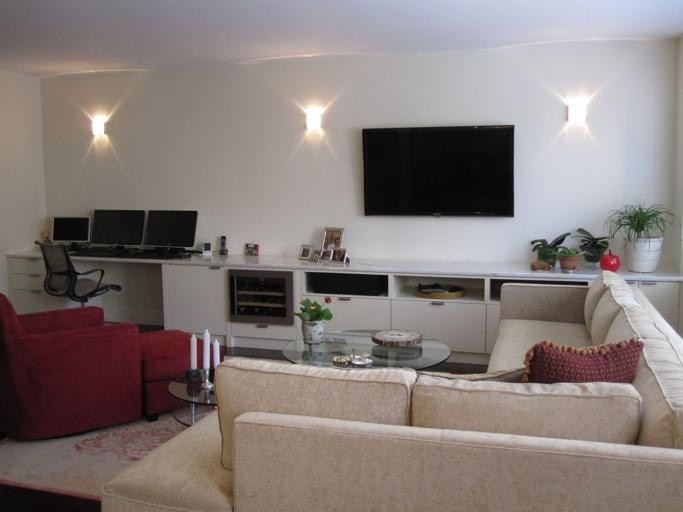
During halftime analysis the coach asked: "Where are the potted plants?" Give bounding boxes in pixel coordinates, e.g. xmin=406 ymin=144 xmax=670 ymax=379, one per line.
xmin=530 ymin=232 xmax=573 ymax=269
xmin=604 ymin=200 xmax=678 ymax=273
xmin=571 ymin=226 xmax=610 ymax=263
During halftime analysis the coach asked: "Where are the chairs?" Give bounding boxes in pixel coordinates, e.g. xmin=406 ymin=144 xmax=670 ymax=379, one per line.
xmin=0 ymin=292 xmax=143 ymax=441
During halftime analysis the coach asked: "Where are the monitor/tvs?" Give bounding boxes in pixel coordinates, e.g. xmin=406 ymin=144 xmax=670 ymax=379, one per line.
xmin=142 ymin=209 xmax=199 ymax=258
xmin=362 ymin=125 xmax=514 ymax=216
xmin=51 ymin=216 xmax=91 ymax=254
xmin=91 ymin=209 xmax=146 ymax=255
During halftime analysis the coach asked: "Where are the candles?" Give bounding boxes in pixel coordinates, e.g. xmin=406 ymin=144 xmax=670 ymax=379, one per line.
xmin=203 ymin=328 xmax=211 ymax=371
xmin=188 ymin=332 xmax=198 ymax=370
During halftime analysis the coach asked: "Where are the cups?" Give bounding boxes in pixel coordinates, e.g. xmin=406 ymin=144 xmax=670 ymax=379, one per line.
xmin=332 ymin=352 xmax=373 ymax=368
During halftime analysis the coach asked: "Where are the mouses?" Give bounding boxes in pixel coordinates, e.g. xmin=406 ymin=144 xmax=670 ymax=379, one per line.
xmin=184 ymin=253 xmax=191 ymax=258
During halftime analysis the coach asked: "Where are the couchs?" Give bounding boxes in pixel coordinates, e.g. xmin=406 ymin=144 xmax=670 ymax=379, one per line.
xmin=99 ymin=267 xmax=683 ymax=511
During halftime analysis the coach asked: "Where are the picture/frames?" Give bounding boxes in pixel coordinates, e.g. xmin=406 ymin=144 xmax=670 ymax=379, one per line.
xmin=321 ymin=227 xmax=344 ymax=249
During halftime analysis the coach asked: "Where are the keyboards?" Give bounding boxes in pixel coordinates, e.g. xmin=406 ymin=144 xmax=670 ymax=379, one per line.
xmin=133 ymin=252 xmax=171 ymax=259
xmin=76 ymin=250 xmax=113 ymax=257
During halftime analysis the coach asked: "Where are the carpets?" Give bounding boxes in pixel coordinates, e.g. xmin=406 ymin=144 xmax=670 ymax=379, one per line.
xmin=0 ymin=401 xmax=217 ymax=502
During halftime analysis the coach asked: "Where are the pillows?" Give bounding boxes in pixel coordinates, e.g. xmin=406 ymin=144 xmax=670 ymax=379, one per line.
xmin=416 ymin=365 xmax=526 ymax=382
xmin=521 ymin=334 xmax=645 ymax=384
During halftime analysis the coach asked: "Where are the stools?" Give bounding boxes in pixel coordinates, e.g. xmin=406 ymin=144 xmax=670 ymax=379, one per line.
xmin=141 ymin=330 xmax=225 ymax=422
